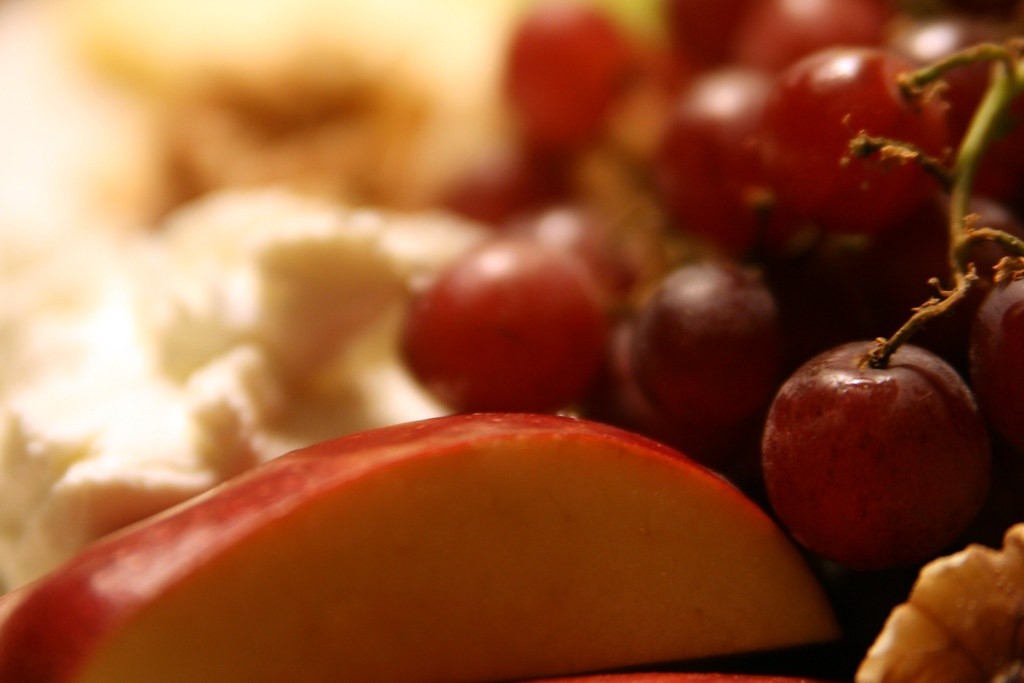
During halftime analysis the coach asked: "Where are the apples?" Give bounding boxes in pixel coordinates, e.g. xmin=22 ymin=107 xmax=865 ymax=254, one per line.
xmin=0 ymin=410 xmax=837 ymax=683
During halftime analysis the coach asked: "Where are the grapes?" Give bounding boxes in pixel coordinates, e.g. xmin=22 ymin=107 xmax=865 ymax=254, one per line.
xmin=389 ymin=0 xmax=1023 ymax=571
